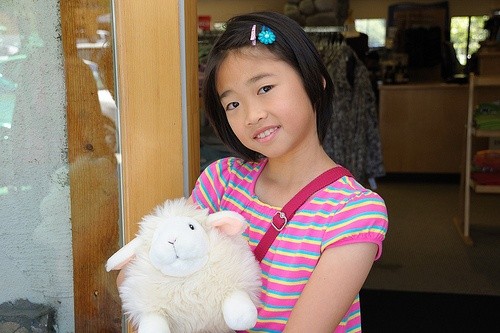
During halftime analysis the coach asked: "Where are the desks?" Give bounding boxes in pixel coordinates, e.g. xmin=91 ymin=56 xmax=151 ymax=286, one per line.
xmin=378 ymin=80 xmax=470 ymax=174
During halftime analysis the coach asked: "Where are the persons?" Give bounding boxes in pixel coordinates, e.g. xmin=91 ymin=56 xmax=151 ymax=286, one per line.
xmin=187 ymin=12 xmax=389 ymax=333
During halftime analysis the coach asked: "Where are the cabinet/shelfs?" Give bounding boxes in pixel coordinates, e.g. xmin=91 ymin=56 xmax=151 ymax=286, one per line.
xmin=459 ymin=71 xmax=500 ymax=238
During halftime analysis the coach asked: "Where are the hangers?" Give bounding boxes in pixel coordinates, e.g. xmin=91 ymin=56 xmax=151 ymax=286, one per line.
xmin=302 ymin=24 xmax=349 ymax=48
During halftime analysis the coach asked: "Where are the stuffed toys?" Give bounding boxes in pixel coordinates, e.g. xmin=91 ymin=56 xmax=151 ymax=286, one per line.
xmin=105 ymin=196 xmax=263 ymax=333
xmin=284 ymin=0 xmax=344 ymax=26
